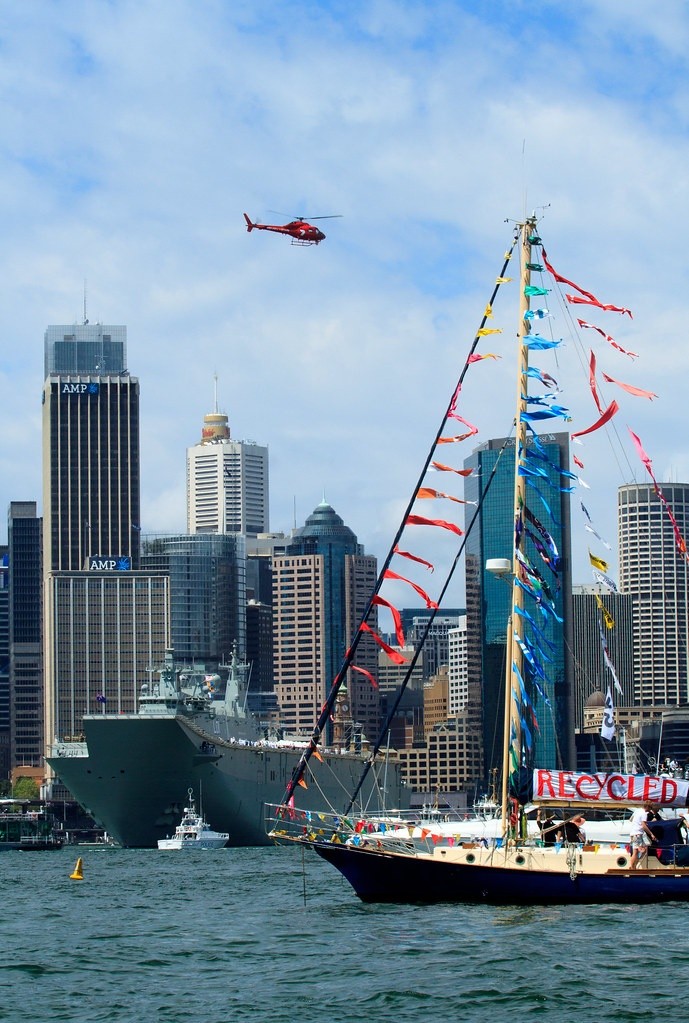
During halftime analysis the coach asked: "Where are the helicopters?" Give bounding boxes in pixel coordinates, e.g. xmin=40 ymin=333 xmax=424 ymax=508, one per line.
xmin=243 ymin=211 xmax=342 ymax=247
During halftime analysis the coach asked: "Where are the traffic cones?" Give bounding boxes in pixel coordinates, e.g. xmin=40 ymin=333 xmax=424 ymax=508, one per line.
xmin=70 ymin=857 xmax=84 ymax=879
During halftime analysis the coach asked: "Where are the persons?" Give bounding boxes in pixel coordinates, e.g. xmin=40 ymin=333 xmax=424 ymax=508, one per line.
xmin=558 ymin=813 xmax=593 ymax=848
xmin=628 ymin=799 xmax=657 ymax=869
xmin=661 ymin=756 xmax=678 ymax=771
xmin=536 ymin=810 xmax=559 ymax=848
xmin=647 ymin=804 xmax=664 ymax=821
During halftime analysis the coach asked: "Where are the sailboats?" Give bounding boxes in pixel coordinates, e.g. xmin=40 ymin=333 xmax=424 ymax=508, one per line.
xmin=266 ymin=138 xmax=689 ymax=904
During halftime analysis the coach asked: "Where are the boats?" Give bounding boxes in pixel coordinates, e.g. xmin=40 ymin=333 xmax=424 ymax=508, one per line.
xmin=158 ymin=780 xmax=228 ymax=850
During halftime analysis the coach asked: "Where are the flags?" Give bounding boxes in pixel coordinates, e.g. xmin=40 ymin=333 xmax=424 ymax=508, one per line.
xmin=207 ymin=681 xmax=216 ymax=693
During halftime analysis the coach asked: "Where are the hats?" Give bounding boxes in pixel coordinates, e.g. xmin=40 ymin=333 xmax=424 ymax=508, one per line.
xmin=570 ymin=816 xmax=586 ymax=826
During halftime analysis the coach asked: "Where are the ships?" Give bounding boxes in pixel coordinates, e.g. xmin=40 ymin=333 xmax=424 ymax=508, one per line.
xmin=42 ymin=636 xmax=414 ymax=849
